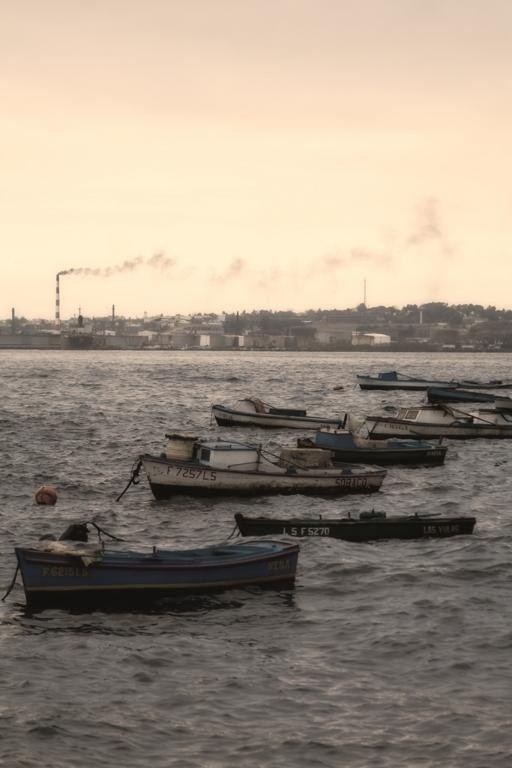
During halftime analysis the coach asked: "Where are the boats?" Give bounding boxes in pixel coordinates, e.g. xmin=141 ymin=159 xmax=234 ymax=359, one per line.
xmin=14 ymin=538 xmax=300 ymax=613
xmin=139 ymin=434 xmax=386 ymax=500
xmin=426 ymin=386 xmax=512 ymax=403
xmin=212 ymin=396 xmax=343 ymax=430
xmin=451 ymin=377 xmax=511 ymax=388
xmin=357 ymin=371 xmax=459 ymax=390
xmin=362 ymin=400 xmax=512 ymax=440
xmin=297 ymin=424 xmax=448 ymax=468
xmin=228 ymin=508 xmax=476 ymax=542
